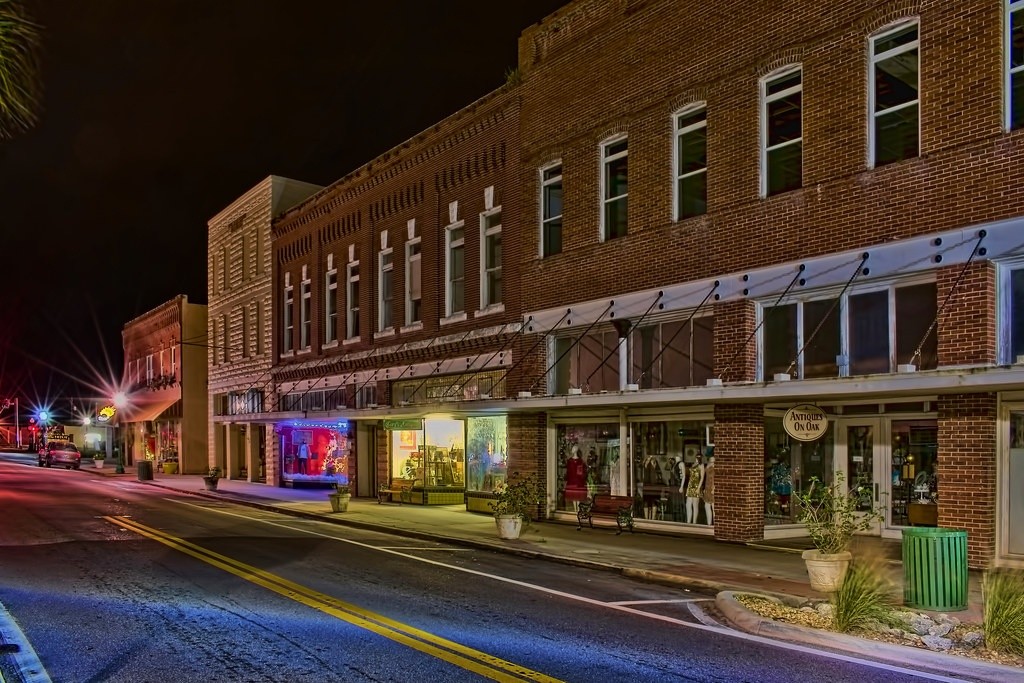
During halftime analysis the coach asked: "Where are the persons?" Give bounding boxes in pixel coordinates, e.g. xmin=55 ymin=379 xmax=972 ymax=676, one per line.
xmin=669 ymin=454 xmax=715 ymax=525
xmin=643 ymin=456 xmax=661 ymax=520
xmin=296 ymin=438 xmax=311 ymax=475
xmin=609 ymin=447 xmax=620 ymax=500
xmin=563 ymin=446 xmax=587 ymax=512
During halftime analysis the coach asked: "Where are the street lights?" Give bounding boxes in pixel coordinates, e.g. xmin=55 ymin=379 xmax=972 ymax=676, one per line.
xmin=110 ymin=393 xmax=128 ymax=473
xmin=39 ymin=411 xmax=47 ymax=447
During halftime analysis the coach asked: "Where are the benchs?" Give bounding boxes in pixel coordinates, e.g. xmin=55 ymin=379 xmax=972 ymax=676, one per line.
xmin=378 ymin=479 xmax=416 ymax=504
xmin=577 ymin=492 xmax=636 ymax=536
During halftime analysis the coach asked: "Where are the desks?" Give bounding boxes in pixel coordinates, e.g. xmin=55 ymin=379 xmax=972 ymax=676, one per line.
xmin=908 ymin=503 xmax=937 ymax=527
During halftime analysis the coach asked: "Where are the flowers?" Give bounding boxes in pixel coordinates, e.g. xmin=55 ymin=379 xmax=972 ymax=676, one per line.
xmin=92 ymin=453 xmax=105 ymax=460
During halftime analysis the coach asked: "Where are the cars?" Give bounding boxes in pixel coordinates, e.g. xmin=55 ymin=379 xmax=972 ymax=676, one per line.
xmin=39 ymin=441 xmax=82 ymax=470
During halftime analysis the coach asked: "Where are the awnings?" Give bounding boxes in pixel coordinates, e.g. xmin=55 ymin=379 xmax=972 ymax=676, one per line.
xmin=121 ymin=398 xmax=180 ymax=422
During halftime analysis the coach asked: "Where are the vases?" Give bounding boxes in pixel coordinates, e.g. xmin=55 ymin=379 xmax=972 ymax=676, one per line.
xmin=162 ymin=462 xmax=178 ymax=475
xmin=94 ymin=460 xmax=104 ymax=468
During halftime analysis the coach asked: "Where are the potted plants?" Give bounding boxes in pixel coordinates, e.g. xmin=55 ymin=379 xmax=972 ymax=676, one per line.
xmin=487 ymin=470 xmax=552 ymax=543
xmin=329 ymin=482 xmax=352 ymax=513
xmin=203 ymin=467 xmax=221 ymax=492
xmin=784 ymin=466 xmax=890 ymax=592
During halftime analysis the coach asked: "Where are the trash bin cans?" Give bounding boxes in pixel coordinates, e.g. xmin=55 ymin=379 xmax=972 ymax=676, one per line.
xmin=900 ymin=525 xmax=969 ymax=612
xmin=137 ymin=460 xmax=153 ymax=480
